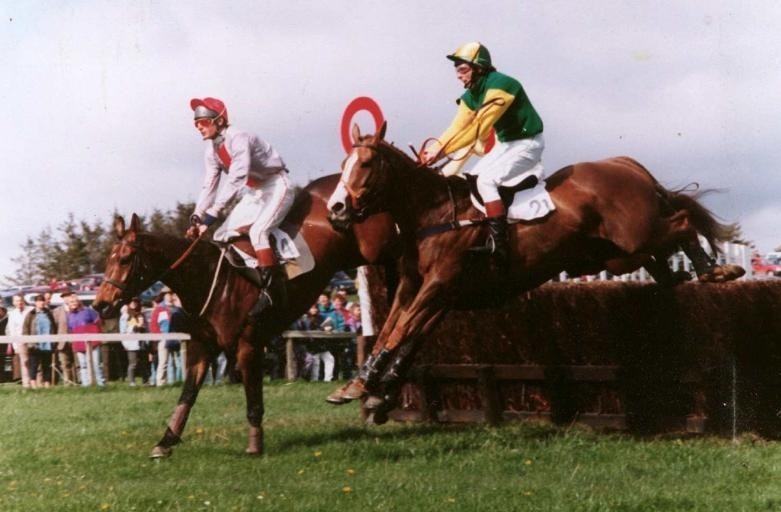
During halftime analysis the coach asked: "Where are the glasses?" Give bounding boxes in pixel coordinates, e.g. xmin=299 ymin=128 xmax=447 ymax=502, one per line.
xmin=194 ymin=108 xmax=226 ymax=127
xmin=457 ymin=66 xmax=470 ymax=74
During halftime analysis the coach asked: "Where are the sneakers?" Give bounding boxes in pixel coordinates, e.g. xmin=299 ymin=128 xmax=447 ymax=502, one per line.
xmin=468 ymin=222 xmax=507 ymax=259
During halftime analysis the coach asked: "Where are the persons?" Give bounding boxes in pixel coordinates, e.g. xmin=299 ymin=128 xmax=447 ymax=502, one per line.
xmin=188 ymin=96 xmax=294 ymax=317
xmin=264 ymin=287 xmax=361 ymax=384
xmin=423 ymin=42 xmax=546 ymax=250
xmin=1 ymin=278 xmax=188 ymax=388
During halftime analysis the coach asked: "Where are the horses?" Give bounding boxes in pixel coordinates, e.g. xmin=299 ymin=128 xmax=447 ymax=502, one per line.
xmin=322 ymin=115 xmax=748 ymax=407
xmin=87 ymin=170 xmax=397 ymax=461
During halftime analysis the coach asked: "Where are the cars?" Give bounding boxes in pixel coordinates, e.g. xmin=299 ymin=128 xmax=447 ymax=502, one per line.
xmin=0 ymin=273 xmax=164 ymax=309
xmin=752 ymin=252 xmax=781 ymax=276
xmin=324 ymin=271 xmax=357 ymax=294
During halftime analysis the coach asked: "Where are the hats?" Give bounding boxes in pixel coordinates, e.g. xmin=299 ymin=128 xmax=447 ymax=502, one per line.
xmin=191 ymin=97 xmax=228 ymax=120
xmin=446 ymin=41 xmax=490 ymax=70
xmin=155 ymin=287 xmax=172 ymax=301
xmin=59 ymin=287 xmax=76 ymax=298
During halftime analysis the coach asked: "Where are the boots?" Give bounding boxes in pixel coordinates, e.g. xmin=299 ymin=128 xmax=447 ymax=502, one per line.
xmin=249 ymin=263 xmax=285 ymax=318
